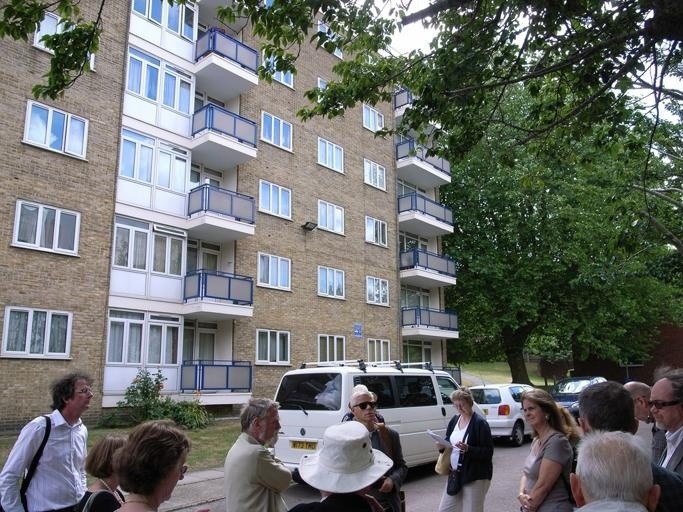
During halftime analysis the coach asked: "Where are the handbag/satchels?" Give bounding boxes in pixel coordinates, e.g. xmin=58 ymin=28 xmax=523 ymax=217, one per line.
xmin=400 ymin=491 xmax=405 ymax=512
xmin=447 ymin=470 xmax=462 ymax=495
xmin=434 ymin=453 xmax=450 ymax=474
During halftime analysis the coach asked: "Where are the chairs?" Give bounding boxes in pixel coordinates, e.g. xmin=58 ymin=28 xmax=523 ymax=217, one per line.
xmin=402 ymin=382 xmax=437 ymax=407
xmin=367 ymin=383 xmax=394 ymax=408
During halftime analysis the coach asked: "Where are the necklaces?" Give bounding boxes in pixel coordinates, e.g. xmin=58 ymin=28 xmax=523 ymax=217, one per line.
xmin=123 ymin=499 xmax=159 ymax=512
xmin=100 ymin=478 xmax=124 ymax=505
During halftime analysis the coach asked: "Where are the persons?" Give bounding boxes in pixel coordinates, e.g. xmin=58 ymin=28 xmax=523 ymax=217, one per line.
xmin=435 ymin=385 xmax=494 ymax=512
xmin=224 ymin=382 xmax=408 ymax=512
xmin=74 ymin=432 xmax=125 ymax=511
xmin=516 ymin=368 xmax=683 ymax=512
xmin=111 ymin=420 xmax=209 ymax=512
xmin=0 ymin=369 xmax=96 ymax=512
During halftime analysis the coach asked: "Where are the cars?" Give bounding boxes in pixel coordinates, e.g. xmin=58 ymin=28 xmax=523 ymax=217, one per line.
xmin=465 ymin=384 xmax=535 ymax=446
xmin=535 ymin=351 xmax=574 ymax=379
xmin=546 ymin=376 xmax=609 ymax=426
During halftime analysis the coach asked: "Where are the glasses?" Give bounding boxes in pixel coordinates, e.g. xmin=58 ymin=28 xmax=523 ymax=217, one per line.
xmin=353 ymin=402 xmax=377 ymax=409
xmin=75 ymin=388 xmax=90 ymax=393
xmin=649 ymin=400 xmax=681 ymax=409
xmin=166 ymin=465 xmax=187 ymax=474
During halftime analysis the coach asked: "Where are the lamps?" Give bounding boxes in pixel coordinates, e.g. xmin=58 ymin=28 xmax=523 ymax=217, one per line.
xmin=301 ymin=222 xmax=318 ymax=231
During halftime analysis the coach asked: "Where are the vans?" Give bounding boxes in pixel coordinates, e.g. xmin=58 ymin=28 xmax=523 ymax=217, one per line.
xmin=266 ymin=359 xmax=485 ymax=490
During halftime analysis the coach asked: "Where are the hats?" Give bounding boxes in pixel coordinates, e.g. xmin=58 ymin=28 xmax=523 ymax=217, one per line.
xmin=298 ymin=421 xmax=394 ymax=493
xmin=348 ymin=384 xmax=378 ymax=404
